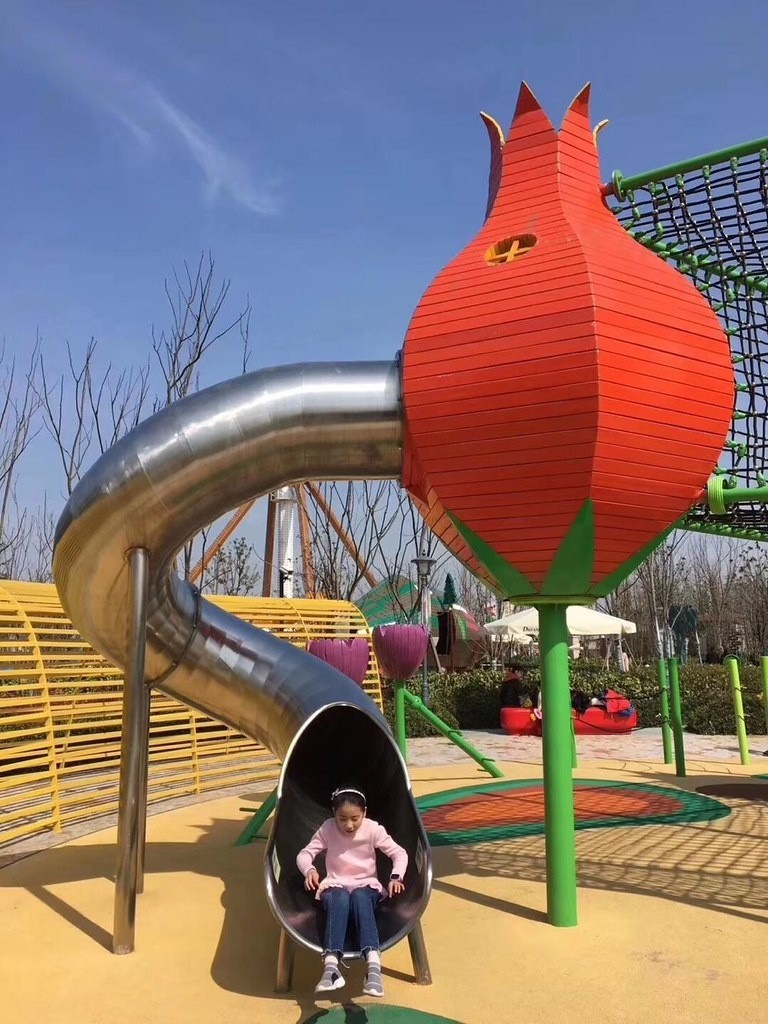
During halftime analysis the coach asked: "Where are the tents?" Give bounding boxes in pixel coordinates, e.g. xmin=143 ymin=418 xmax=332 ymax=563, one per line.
xmin=484 ymin=602 xmax=637 ymax=672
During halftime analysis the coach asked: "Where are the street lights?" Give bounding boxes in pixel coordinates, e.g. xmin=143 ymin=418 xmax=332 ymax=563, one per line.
xmin=411 ymin=548 xmax=437 ymax=710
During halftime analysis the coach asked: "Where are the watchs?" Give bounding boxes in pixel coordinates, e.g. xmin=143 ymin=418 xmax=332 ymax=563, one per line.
xmin=389 ymin=874 xmax=401 ymax=881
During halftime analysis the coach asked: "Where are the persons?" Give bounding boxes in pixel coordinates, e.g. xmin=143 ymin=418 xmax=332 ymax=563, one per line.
xmin=298 ymin=787 xmax=407 ymax=997
xmin=706 ymin=646 xmax=730 ymax=665
xmin=500 ymin=665 xmax=543 ymax=738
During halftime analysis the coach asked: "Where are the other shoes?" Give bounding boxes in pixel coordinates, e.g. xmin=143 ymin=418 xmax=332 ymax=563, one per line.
xmin=362 ymin=962 xmax=385 ymax=997
xmin=314 ymin=963 xmax=346 ymax=992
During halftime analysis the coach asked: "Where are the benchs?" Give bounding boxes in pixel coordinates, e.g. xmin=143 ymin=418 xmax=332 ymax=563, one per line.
xmin=499 ymin=706 xmax=639 ymax=735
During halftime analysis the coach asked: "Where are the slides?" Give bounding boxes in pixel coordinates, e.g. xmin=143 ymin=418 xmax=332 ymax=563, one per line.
xmin=49 ymin=360 xmax=437 ymax=962
